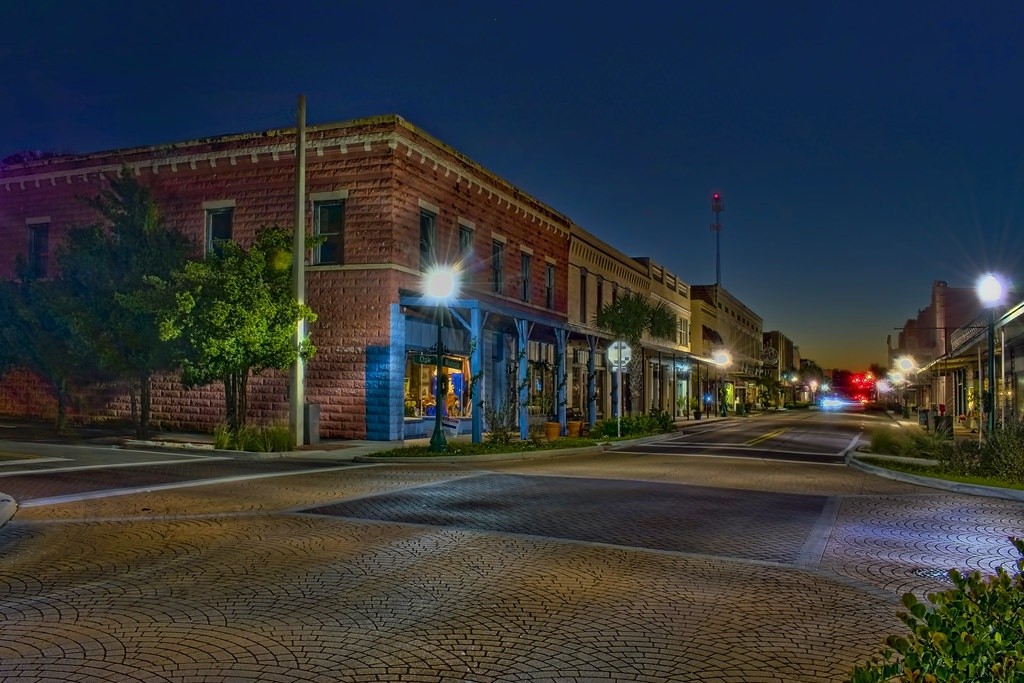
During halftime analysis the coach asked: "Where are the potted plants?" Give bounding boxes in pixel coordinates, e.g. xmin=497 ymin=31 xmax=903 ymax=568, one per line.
xmin=544 ymin=404 xmax=560 ymax=441
xmin=691 ymin=401 xmax=701 ymax=420
xmin=745 ymin=400 xmax=752 ymax=413
xmin=677 ymin=396 xmax=686 ymax=416
xmin=566 ymin=412 xmax=584 ymax=437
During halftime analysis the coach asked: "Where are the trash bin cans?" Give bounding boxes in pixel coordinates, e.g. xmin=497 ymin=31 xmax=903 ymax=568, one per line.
xmin=745 ymin=403 xmax=752 ymax=413
xmin=903 ymin=407 xmax=909 ymax=418
xmin=305 ymin=404 xmax=320 ymax=444
xmin=934 ymin=415 xmax=953 ymax=438
xmin=736 ymin=403 xmax=745 ymax=415
xmin=919 ymin=409 xmax=929 ymax=424
